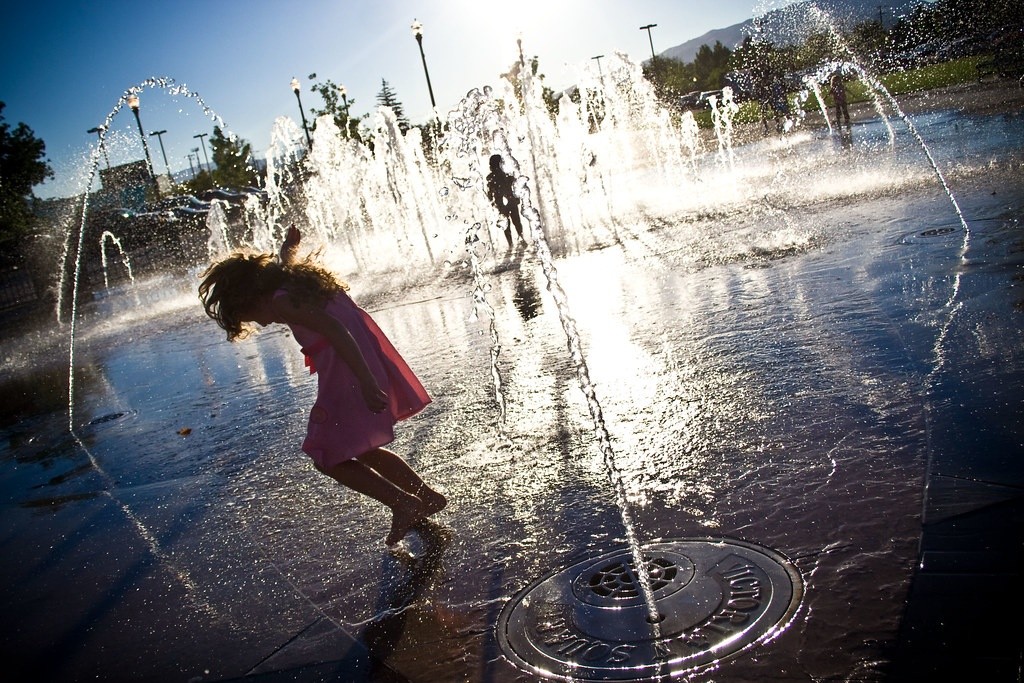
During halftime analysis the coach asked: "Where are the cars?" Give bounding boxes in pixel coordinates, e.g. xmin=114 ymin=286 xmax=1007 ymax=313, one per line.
xmin=70 ymin=185 xmax=270 ymax=252
xmin=679 ymin=38 xmax=976 ymax=110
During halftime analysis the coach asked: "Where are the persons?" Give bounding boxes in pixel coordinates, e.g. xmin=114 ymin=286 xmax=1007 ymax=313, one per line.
xmin=486 ymin=154 xmax=525 ymax=245
xmin=197 ymin=225 xmax=447 ymax=546
xmin=823 ymin=74 xmax=859 ymax=125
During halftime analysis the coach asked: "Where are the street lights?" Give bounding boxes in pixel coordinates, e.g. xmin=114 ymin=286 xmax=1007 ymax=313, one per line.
xmin=339 ymin=83 xmax=349 ymax=116
xmin=591 ymin=55 xmax=606 ymax=84
xmin=125 ymin=89 xmax=158 ymax=181
xmin=639 ymin=22 xmax=660 ymax=71
xmin=290 ymin=76 xmax=314 ymax=152
xmin=148 ymin=130 xmax=172 ymax=180
xmin=184 ymin=154 xmax=195 ymax=178
xmin=86 ymin=125 xmax=121 ymax=209
xmin=189 ymin=148 xmax=203 ymax=170
xmin=408 ymin=17 xmax=436 ymax=108
xmin=194 ymin=133 xmax=210 ymax=171
